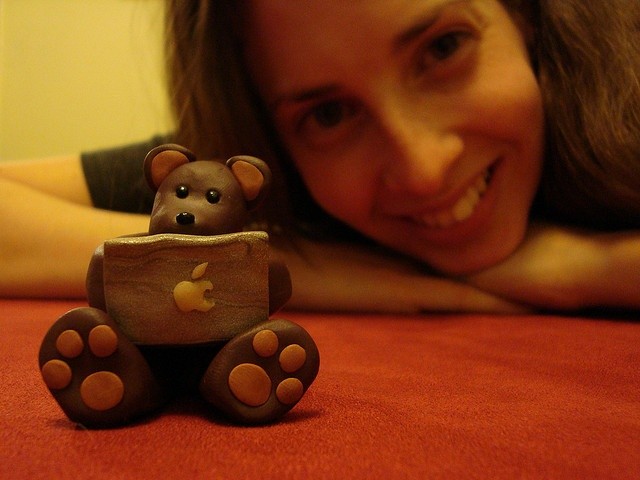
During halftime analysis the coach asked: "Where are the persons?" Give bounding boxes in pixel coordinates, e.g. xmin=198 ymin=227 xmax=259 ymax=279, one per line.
xmin=1 ymin=1 xmax=640 ymax=319
